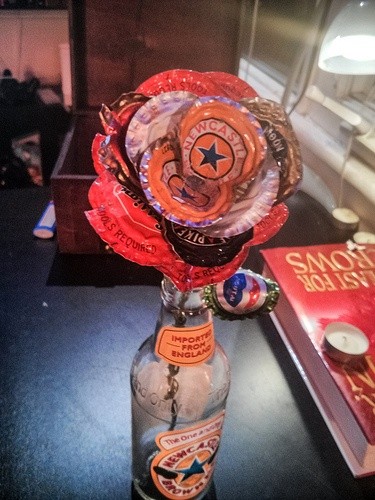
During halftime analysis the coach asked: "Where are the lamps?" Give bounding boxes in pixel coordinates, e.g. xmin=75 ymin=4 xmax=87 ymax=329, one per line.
xmin=318 ymin=0 xmax=375 ymax=77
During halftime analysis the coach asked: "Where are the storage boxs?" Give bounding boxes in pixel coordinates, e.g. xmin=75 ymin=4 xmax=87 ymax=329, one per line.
xmin=50 ymin=109 xmax=125 ymax=257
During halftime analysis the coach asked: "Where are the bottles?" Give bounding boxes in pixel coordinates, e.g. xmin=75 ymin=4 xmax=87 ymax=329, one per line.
xmin=130 ymin=277 xmax=231 ymax=500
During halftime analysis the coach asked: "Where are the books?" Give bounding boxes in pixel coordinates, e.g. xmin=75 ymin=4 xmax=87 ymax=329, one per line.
xmin=260 ymin=243 xmax=375 ymax=478
